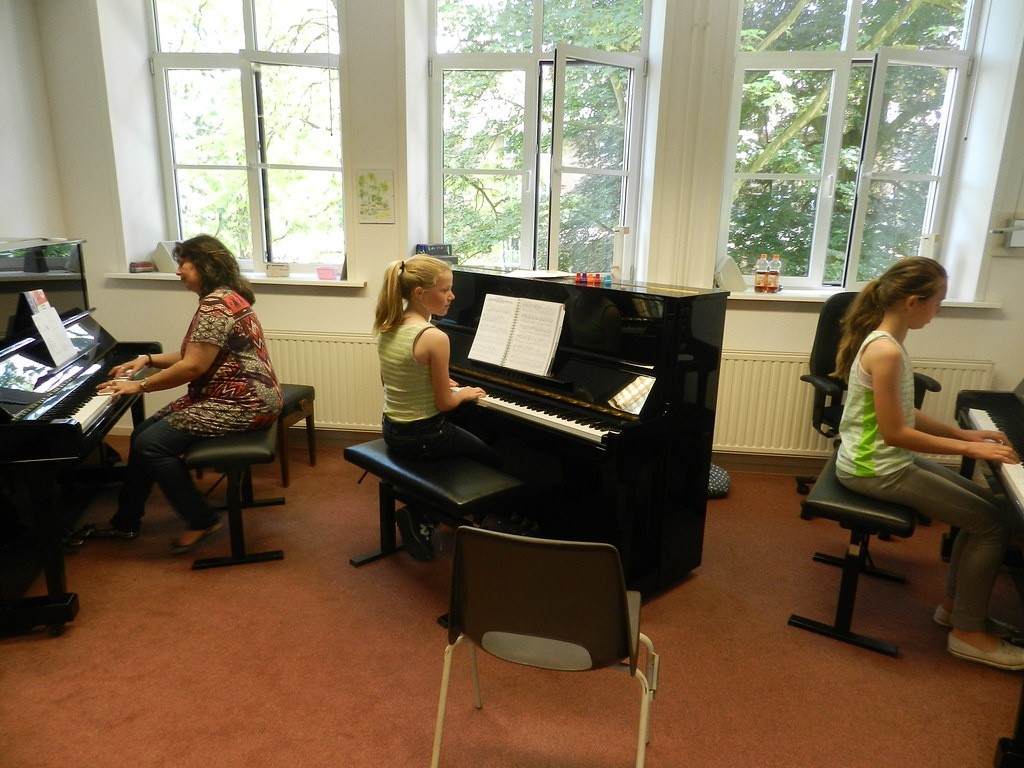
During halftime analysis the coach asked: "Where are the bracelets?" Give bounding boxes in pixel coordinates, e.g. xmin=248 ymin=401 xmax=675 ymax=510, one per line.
xmin=145 ymin=352 xmax=151 ymax=366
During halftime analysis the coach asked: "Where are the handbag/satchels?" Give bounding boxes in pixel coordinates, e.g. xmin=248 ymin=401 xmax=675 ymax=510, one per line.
xmin=708 ymin=464 xmax=730 ymax=497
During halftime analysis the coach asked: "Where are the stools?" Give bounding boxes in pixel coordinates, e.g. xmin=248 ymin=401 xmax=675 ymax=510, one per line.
xmin=346 ymin=437 xmax=524 ymax=629
xmin=265 ymin=383 xmax=316 ymax=487
xmin=184 ymin=420 xmax=286 ymax=571
xmin=787 ymin=441 xmax=915 ymax=658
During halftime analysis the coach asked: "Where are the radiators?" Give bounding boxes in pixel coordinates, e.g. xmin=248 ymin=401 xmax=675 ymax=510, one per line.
xmin=712 ymin=348 xmax=995 ymax=470
xmin=260 ymin=330 xmax=385 ymax=433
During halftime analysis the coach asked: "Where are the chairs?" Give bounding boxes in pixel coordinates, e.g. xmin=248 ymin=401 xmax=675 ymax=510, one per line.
xmin=800 ymin=292 xmax=941 ymax=540
xmin=430 ymin=523 xmax=659 ymax=768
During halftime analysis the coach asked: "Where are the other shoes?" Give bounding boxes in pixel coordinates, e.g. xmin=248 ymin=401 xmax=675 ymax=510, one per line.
xmin=170 ymin=520 xmax=224 ymax=553
xmin=84 ymin=522 xmax=135 ymax=538
xmin=933 ymin=605 xmax=1016 ymax=638
xmin=947 ymin=628 xmax=1024 ymax=671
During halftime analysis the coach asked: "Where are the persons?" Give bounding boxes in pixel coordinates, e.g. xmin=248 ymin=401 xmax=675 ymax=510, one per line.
xmin=566 ymin=287 xmax=621 ymax=373
xmin=836 ymin=257 xmax=1024 ymax=671
xmin=376 ymin=253 xmax=487 ymax=562
xmin=95 ymin=234 xmax=283 ymax=557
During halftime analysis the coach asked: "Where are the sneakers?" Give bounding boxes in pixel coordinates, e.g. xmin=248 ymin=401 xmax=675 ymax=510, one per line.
xmin=397 ymin=507 xmax=436 ymax=561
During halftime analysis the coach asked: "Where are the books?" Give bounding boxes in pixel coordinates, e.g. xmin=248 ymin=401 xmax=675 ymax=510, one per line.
xmin=469 ymin=292 xmax=565 ymax=376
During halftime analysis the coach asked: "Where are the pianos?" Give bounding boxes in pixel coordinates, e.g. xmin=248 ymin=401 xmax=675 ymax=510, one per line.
xmin=940 ymin=378 xmax=1024 ymax=768
xmin=0 ymin=237 xmax=162 ymax=638
xmin=430 ymin=264 xmax=730 ymax=607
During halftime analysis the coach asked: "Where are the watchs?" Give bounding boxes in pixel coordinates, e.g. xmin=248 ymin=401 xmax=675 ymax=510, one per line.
xmin=141 ymin=378 xmax=150 ymax=393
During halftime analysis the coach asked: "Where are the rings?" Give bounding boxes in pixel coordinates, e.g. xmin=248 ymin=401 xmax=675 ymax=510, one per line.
xmin=121 ymin=367 xmax=126 ymax=373
xmin=113 ymin=381 xmax=116 ymax=385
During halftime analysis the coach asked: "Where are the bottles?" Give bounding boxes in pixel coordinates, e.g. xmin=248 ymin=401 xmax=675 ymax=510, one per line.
xmin=754 ymin=254 xmax=768 ymax=292
xmin=767 ymin=254 xmax=781 ymax=293
